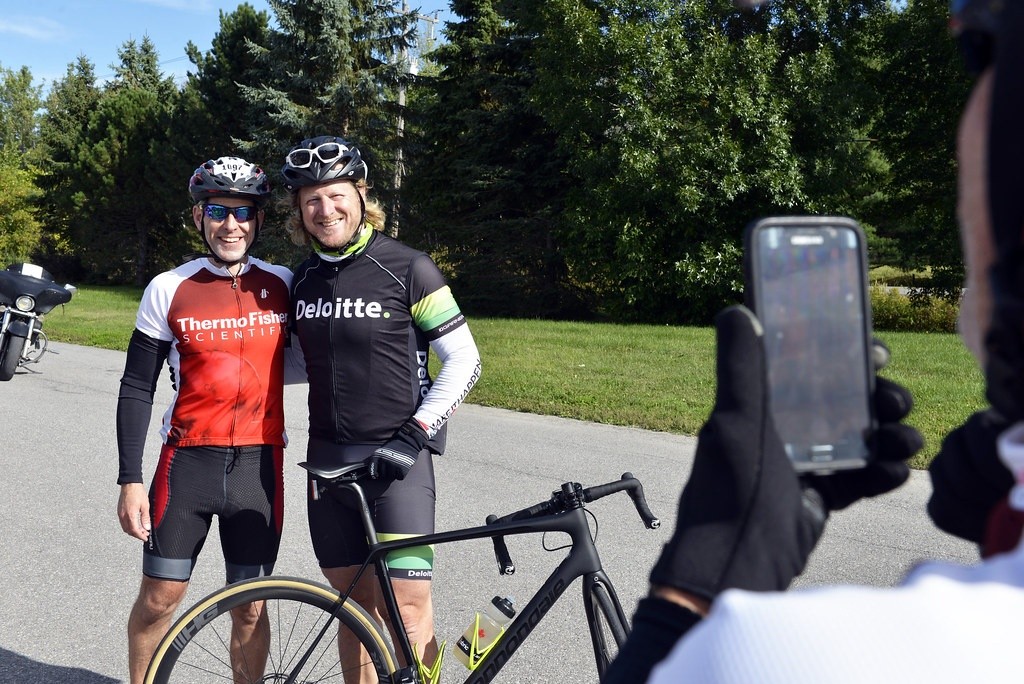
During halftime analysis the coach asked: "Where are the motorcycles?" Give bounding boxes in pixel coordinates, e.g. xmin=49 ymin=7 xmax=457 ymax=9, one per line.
xmin=0 ymin=261 xmax=78 ymax=382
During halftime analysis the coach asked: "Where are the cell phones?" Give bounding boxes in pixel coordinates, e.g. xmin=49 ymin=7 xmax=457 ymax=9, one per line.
xmin=745 ymin=215 xmax=882 ymax=473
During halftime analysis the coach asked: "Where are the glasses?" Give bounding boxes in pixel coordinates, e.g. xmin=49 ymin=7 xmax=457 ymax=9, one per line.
xmin=286 ymin=142 xmax=350 ymax=168
xmin=199 ymin=203 xmax=258 ymax=223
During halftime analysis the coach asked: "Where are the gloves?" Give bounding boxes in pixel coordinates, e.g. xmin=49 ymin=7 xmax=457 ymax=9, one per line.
xmin=646 ymin=302 xmax=923 ymax=600
xmin=369 ymin=415 xmax=429 ymax=485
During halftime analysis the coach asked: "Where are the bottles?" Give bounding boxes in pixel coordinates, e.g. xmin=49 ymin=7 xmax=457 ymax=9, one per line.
xmin=454 ymin=598 xmax=516 ymax=669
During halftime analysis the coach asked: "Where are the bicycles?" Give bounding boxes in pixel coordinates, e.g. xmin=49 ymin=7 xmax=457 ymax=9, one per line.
xmin=143 ymin=460 xmax=659 ymax=684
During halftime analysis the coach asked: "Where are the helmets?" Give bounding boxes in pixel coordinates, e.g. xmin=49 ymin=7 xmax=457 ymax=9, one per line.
xmin=280 ymin=135 xmax=367 ymax=194
xmin=189 ymin=156 xmax=272 ymax=209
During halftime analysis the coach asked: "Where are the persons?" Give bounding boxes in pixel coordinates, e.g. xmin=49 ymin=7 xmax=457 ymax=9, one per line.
xmin=116 ymin=156 xmax=294 ymax=684
xmin=166 ymin=136 xmax=481 ymax=684
xmin=600 ymin=0 xmax=1024 ymax=684
